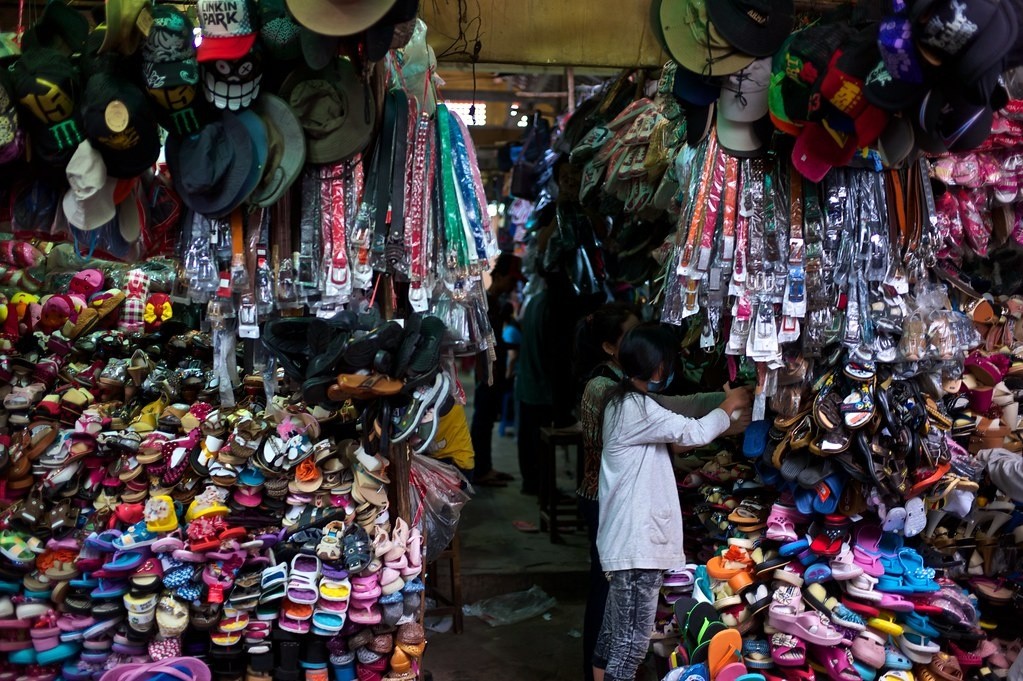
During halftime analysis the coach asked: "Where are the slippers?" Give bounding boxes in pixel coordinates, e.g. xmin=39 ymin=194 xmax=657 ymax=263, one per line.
xmin=655 ymin=366 xmax=1022 ymax=681
xmin=0 ymin=238 xmax=457 ymax=681
xmin=512 ymin=518 xmax=540 ymax=531
xmin=471 ymin=478 xmax=507 ymax=487
xmin=485 ymin=472 xmax=516 ymax=481
xmin=941 ymin=150 xmax=1023 ymax=365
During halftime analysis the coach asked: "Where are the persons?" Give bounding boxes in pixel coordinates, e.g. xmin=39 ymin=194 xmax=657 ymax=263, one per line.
xmin=468 ymin=222 xmax=585 ymax=504
xmin=575 ymin=303 xmax=754 ymax=681
xmin=971 ymin=448 xmax=1023 ymax=681
xmin=411 ymin=391 xmax=476 ymax=489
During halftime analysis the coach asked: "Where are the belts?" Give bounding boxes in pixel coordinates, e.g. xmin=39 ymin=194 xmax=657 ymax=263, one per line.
xmin=661 ymin=131 xmax=940 ymax=364
xmin=184 ymin=104 xmax=501 ymax=384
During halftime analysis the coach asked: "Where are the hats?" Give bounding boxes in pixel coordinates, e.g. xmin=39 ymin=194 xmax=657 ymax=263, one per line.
xmin=659 ymin=0 xmax=1022 ymax=185
xmin=0 ymin=1 xmax=403 ymax=231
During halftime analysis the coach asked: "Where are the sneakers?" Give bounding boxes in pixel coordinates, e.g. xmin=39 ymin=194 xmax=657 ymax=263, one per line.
xmin=506 ymin=68 xmax=682 ymax=316
